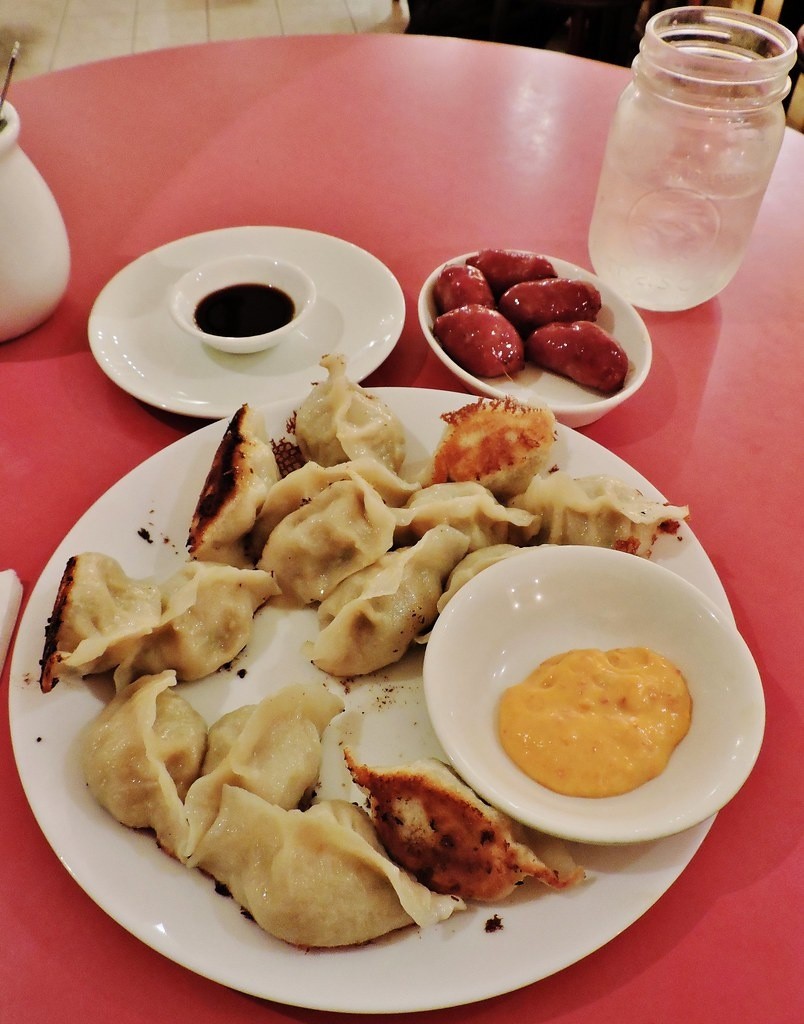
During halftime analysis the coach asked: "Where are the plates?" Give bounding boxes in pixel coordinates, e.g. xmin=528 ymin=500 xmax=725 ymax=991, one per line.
xmin=8 ymin=385 xmax=736 ymax=1015
xmin=87 ymin=224 xmax=407 ymax=419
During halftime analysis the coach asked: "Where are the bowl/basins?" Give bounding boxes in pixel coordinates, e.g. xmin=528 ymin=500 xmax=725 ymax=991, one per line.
xmin=170 ymin=254 xmax=314 ymax=354
xmin=417 ymin=249 xmax=653 ymax=429
xmin=421 ymin=544 xmax=768 ymax=847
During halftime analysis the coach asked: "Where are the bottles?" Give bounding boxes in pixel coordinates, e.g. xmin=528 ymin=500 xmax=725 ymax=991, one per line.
xmin=587 ymin=7 xmax=798 ymax=312
xmin=0 ymin=98 xmax=71 ymax=344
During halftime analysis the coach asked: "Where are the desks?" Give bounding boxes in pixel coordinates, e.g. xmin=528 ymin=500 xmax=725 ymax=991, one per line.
xmin=0 ymin=36 xmax=804 ymax=1024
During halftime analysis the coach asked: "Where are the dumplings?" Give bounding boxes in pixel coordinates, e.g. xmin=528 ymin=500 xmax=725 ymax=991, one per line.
xmin=39 ymin=349 xmax=689 ymax=949
